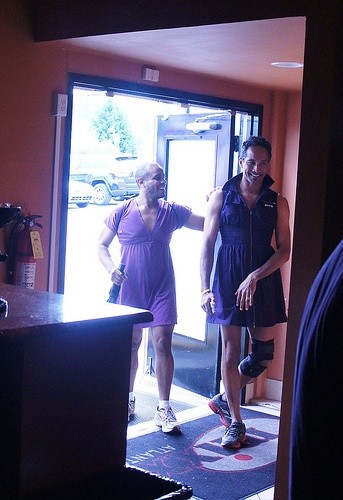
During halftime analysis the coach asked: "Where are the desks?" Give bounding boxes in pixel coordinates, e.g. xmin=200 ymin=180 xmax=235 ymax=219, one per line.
xmin=0 ymin=282 xmax=154 ymax=500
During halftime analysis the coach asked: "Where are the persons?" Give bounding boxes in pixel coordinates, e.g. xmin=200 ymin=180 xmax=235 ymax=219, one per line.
xmin=201 ymin=136 xmax=290 ymax=447
xmin=98 ymin=162 xmax=220 ymax=433
xmin=290 ymin=238 xmax=343 ymax=500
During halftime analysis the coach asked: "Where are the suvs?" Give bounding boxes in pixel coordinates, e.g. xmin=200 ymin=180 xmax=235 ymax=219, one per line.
xmin=69 ymin=153 xmax=145 ymax=205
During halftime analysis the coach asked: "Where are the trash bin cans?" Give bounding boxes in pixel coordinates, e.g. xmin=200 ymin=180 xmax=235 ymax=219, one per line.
xmin=53 ymin=460 xmax=191 ymax=500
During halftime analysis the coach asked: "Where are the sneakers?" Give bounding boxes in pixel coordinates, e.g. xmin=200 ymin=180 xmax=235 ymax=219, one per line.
xmin=128 ymin=396 xmax=135 ymax=422
xmin=220 ymin=422 xmax=246 ymax=448
xmin=154 ymin=405 xmax=182 ymax=434
xmin=208 ymin=393 xmax=232 ymax=428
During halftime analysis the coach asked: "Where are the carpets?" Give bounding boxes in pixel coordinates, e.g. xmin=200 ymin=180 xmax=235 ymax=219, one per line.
xmin=126 ymin=405 xmax=280 ymax=500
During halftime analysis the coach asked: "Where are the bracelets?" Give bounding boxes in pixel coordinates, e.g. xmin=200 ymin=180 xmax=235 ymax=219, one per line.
xmin=202 ymin=289 xmax=211 ymax=295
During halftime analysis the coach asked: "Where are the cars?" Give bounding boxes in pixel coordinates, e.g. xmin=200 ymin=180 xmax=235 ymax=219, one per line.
xmin=69 ymin=180 xmax=95 ymax=209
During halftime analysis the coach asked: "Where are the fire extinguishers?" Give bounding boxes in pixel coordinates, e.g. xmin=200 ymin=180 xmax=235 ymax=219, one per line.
xmin=7 ymin=214 xmax=43 ymax=290
